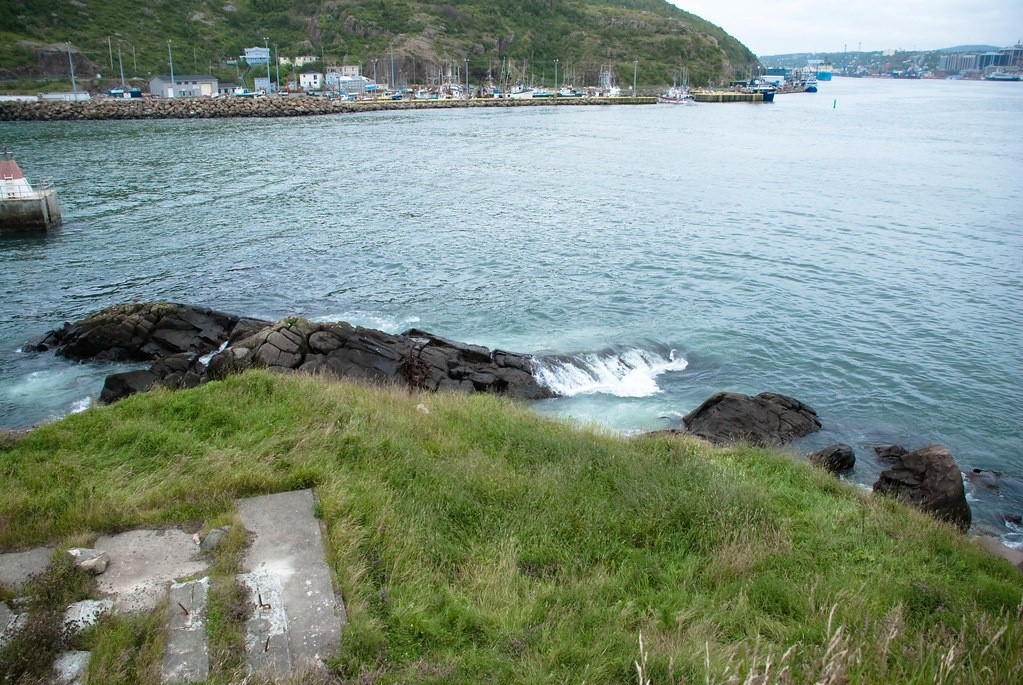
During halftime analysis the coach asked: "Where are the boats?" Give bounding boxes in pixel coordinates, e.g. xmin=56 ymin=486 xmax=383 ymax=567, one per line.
xmin=658 ymin=95 xmax=687 ymax=103
xmin=235 ymin=92 xmax=262 ymax=98
xmin=556 ymin=93 xmax=583 ymax=97
xmin=985 ymin=71 xmax=1023 ymax=81
xmin=533 ymin=92 xmax=554 ymax=97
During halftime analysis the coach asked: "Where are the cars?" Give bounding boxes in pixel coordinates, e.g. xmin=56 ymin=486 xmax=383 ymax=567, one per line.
xmin=278 ymin=90 xmax=289 ymax=96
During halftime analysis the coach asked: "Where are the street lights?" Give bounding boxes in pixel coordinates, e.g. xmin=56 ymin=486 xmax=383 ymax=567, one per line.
xmin=389 ymin=42 xmax=394 ymax=88
xmin=633 ymin=60 xmax=638 ymax=96
xmin=166 ymin=39 xmax=177 ymax=97
xmin=263 ymin=36 xmax=271 ymax=94
xmin=464 ymin=58 xmax=469 ymax=97
xmin=66 ymin=41 xmax=78 ymax=101
xmin=553 ymin=58 xmax=559 ymax=98
xmin=273 ymin=43 xmax=280 ymax=91
xmin=117 ymin=43 xmax=124 ymax=87
